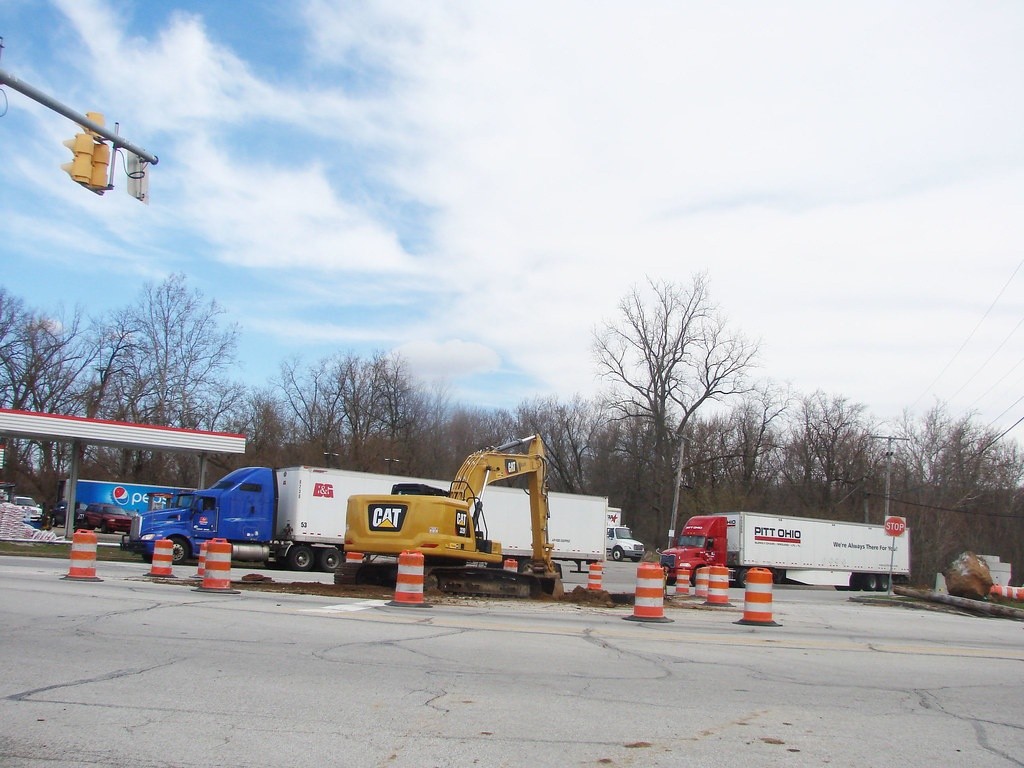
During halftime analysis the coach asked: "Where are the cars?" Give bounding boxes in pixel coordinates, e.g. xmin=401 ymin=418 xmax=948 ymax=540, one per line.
xmin=84 ymin=503 xmax=133 ymax=535
xmin=13 ymin=496 xmax=43 ymax=522
xmin=50 ymin=501 xmax=88 ymax=530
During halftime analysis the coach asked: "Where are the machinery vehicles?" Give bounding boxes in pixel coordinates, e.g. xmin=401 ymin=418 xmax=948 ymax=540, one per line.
xmin=334 ymin=432 xmax=565 ymax=600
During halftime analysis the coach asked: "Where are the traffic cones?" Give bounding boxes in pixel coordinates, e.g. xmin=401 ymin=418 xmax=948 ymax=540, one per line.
xmin=677 ymin=564 xmax=731 ymax=606
xmin=346 ymin=552 xmax=364 ymax=566
xmin=623 ymin=562 xmax=674 ymax=624
xmin=58 ymin=529 xmax=105 ymax=582
xmin=587 ymin=564 xmax=605 ymax=590
xmin=735 ymin=566 xmax=782 ymax=627
xmin=383 ymin=549 xmax=435 ymax=608
xmin=142 ymin=539 xmax=179 ymax=578
xmin=188 ymin=536 xmax=242 ymax=594
xmin=502 ymin=558 xmax=518 ymax=574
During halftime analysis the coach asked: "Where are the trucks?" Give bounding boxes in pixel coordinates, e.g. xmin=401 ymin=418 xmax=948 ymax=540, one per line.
xmin=660 ymin=511 xmax=912 ymax=592
xmin=120 ymin=465 xmax=606 ymax=583
xmin=605 ymin=508 xmax=645 ymax=562
